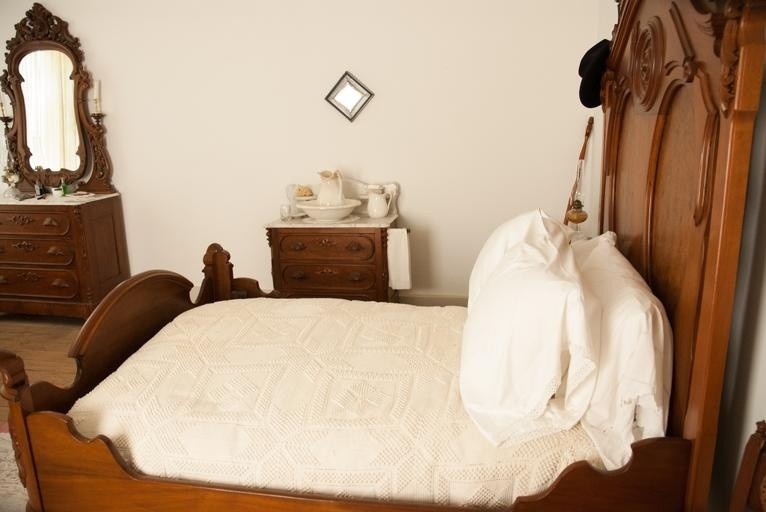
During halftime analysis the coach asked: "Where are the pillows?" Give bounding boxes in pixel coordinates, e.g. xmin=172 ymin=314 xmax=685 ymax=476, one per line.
xmin=565 ymin=224 xmax=672 ymax=464
xmin=461 ymin=213 xmax=601 ymax=448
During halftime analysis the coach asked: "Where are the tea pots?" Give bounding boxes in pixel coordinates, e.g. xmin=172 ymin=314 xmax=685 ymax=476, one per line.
xmin=316 ymin=170 xmax=346 ymax=207
xmin=367 ymin=185 xmax=393 ymax=218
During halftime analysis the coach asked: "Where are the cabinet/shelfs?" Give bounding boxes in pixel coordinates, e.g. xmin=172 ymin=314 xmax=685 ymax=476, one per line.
xmin=0 ymin=192 xmax=131 ymax=323
xmin=263 ymin=212 xmax=399 ymax=300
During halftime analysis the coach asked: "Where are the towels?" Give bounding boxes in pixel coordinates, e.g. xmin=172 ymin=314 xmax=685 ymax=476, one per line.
xmin=386 ymin=228 xmax=412 ymax=291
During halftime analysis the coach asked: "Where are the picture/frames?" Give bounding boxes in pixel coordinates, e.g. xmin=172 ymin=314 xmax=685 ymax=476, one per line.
xmin=325 ymin=71 xmax=373 ymax=121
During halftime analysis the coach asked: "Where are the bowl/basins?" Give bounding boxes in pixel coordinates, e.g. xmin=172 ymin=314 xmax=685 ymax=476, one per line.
xmin=296 ymin=198 xmax=361 ymax=222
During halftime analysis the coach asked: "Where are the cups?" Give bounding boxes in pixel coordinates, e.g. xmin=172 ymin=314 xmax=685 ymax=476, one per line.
xmin=279 ymin=204 xmax=291 ymax=225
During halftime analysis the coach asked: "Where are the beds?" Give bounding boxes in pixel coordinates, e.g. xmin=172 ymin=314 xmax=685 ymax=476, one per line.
xmin=0 ymin=1 xmax=765 ymax=510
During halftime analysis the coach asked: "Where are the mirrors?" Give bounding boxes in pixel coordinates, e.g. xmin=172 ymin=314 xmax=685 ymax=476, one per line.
xmin=0 ymin=2 xmax=115 ymax=197
